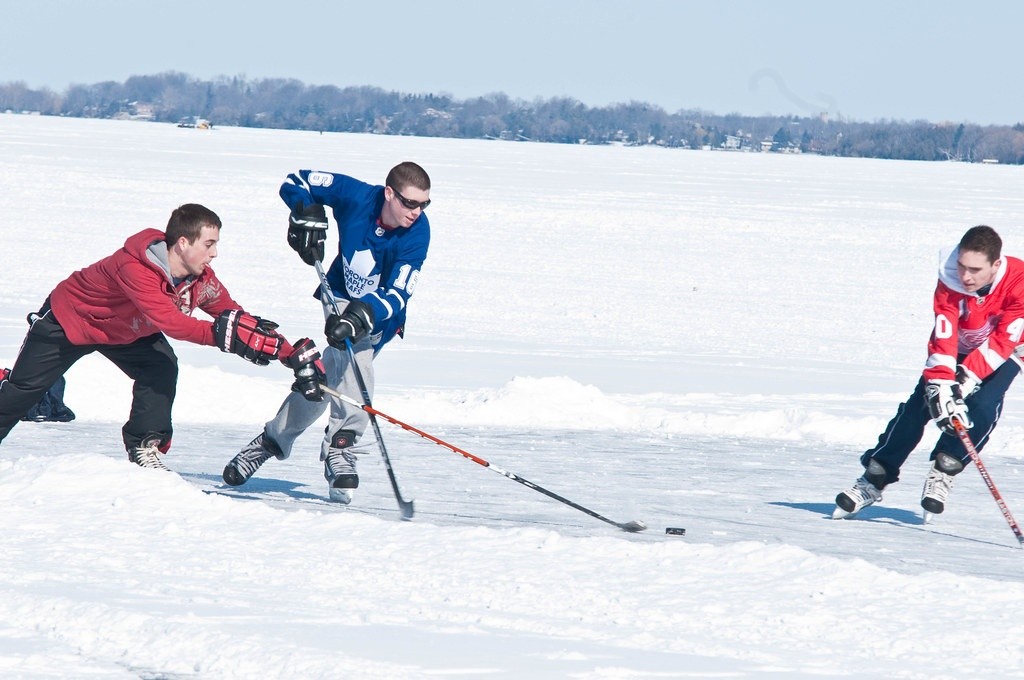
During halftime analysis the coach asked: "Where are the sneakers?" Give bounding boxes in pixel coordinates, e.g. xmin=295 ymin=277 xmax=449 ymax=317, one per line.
xmin=128 ymin=434 xmax=172 ymax=471
xmin=832 ymin=459 xmax=888 ymax=519
xmin=325 ymin=426 xmax=378 ymax=504
xmin=921 ymin=453 xmax=964 ymax=525
xmin=223 ymin=426 xmax=280 ymax=487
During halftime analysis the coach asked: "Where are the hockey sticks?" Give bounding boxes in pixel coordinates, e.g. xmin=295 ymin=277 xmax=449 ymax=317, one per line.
xmin=311 ymin=243 xmax=415 ymax=519
xmin=319 ymin=382 xmax=647 ymax=534
xmin=952 ymin=417 xmax=1024 ymax=544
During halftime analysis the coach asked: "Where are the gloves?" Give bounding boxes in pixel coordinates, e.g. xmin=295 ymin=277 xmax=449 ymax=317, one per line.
xmin=281 ymin=337 xmax=328 ymax=402
xmin=923 ymin=383 xmax=974 ymax=438
xmin=953 ymin=364 xmax=983 ymax=400
xmin=214 ymin=309 xmax=284 ymax=366
xmin=288 ymin=204 xmax=329 ymax=266
xmin=324 ymin=300 xmax=374 ymax=352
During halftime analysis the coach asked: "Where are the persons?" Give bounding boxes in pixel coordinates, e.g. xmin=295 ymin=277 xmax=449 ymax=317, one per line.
xmin=219 ymin=162 xmax=431 ymax=504
xmin=0 ymin=203 xmax=328 ymax=472
xmin=832 ymin=226 xmax=1024 ymax=525
xmin=202 ymin=122 xmax=214 ymax=130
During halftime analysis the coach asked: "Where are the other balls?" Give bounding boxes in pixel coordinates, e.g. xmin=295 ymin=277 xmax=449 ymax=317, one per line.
xmin=665 ymin=528 xmax=685 ymax=535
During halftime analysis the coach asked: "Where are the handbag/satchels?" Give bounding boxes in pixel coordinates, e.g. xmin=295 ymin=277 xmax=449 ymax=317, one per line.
xmin=0 ymin=368 xmax=76 ymax=423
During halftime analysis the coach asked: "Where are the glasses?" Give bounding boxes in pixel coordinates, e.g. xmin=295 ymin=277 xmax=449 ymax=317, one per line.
xmin=390 ymin=187 xmax=431 ymax=210
